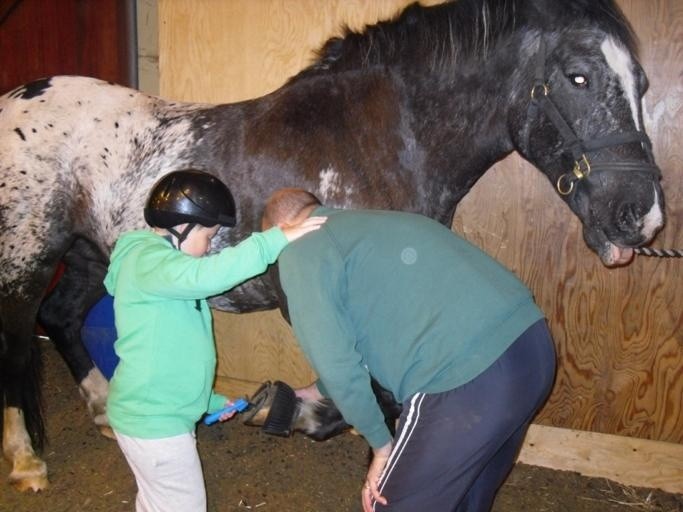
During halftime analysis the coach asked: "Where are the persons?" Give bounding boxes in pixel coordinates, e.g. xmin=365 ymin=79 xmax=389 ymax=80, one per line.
xmin=106 ymin=167 xmax=327 ymax=512
xmin=261 ymin=189 xmax=558 ymax=512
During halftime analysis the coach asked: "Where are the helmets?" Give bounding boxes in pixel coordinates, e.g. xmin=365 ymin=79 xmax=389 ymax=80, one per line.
xmin=145 ymin=170 xmax=237 ymax=227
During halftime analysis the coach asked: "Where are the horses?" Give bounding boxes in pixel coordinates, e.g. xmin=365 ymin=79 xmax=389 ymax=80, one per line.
xmin=0 ymin=0 xmax=668 ymax=495
